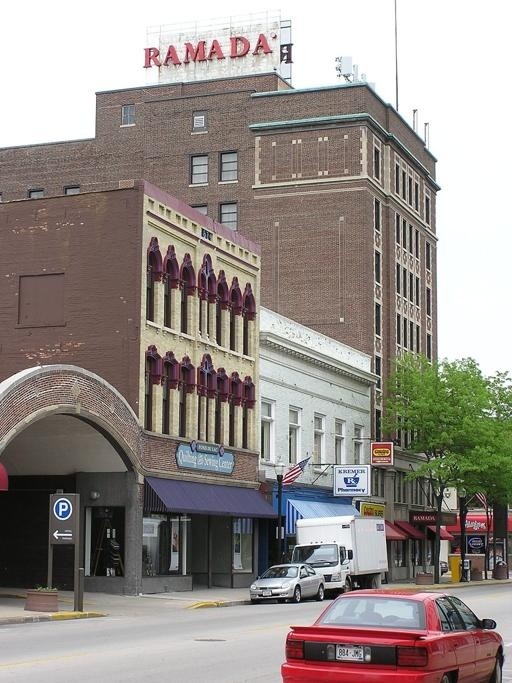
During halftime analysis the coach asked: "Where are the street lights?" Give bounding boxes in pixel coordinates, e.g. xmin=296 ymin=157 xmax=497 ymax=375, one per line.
xmin=273 ymin=456 xmax=287 ymax=563
xmin=458 ymin=484 xmax=467 ymax=582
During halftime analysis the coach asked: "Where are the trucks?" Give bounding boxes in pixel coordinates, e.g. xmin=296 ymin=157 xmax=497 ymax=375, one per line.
xmin=291 ymin=515 xmax=389 ymax=594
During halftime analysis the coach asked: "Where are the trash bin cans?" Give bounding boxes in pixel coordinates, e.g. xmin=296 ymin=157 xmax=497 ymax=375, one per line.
xmin=495 ymin=561 xmax=508 ymax=579
xmin=461 ymin=559 xmax=471 ymax=582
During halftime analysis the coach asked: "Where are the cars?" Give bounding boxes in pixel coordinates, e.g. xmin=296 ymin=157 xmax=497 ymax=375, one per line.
xmin=249 ymin=564 xmax=326 ymax=603
xmin=281 ymin=588 xmax=505 ymax=682
xmin=419 ymin=555 xmax=447 ymax=575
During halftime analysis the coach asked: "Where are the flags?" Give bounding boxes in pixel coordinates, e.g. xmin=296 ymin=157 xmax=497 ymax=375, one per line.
xmin=282 ymin=456 xmax=310 ymax=485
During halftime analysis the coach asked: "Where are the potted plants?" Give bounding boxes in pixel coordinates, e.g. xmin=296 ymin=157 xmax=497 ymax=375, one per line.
xmin=25 ymin=584 xmax=59 ymax=612
